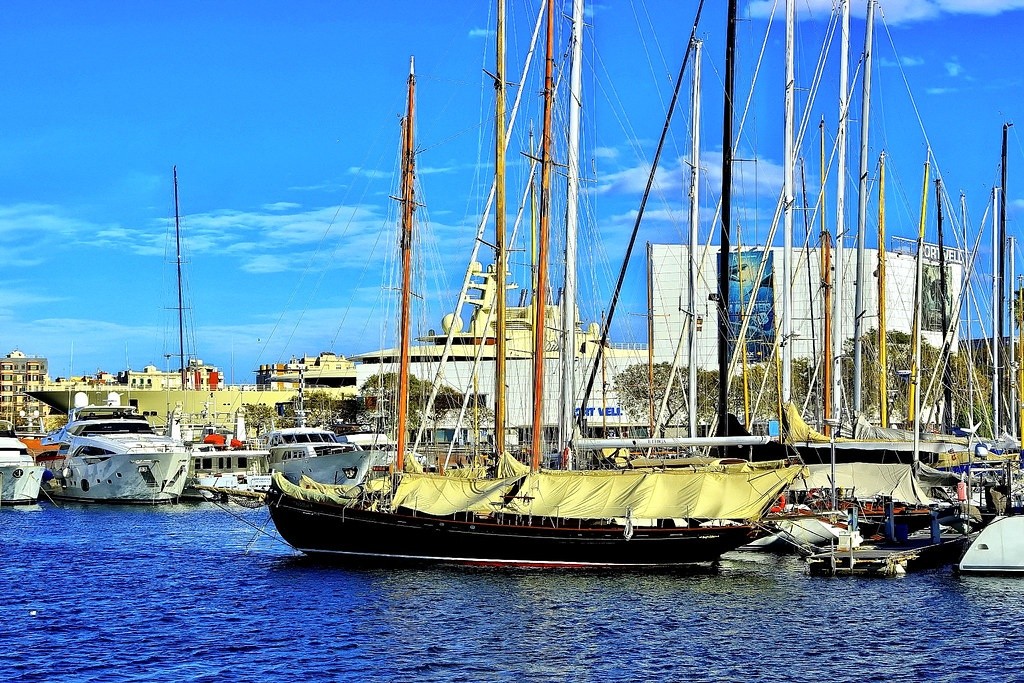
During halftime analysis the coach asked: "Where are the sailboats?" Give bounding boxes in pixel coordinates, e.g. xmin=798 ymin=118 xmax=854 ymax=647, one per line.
xmin=263 ymin=0 xmax=1021 ymax=579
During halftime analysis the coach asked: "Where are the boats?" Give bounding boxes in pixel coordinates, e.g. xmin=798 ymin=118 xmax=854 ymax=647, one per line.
xmin=34 ymin=404 xmax=188 ymax=503
xmin=165 ymin=398 xmax=428 ymax=507
xmin=0 ymin=418 xmax=46 ymax=505
xmin=24 ymin=164 xmax=358 ymax=425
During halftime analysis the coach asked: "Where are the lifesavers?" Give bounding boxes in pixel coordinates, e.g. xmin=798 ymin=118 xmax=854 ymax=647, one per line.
xmin=563 ymin=446 xmax=571 ymax=467
xmin=770 ymin=495 xmax=785 ymax=512
